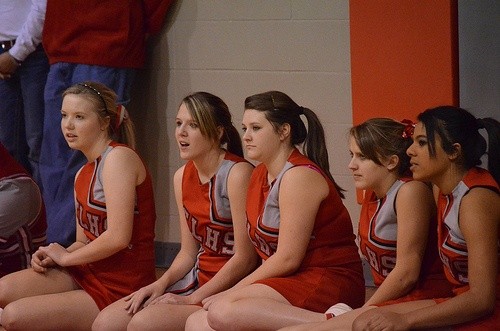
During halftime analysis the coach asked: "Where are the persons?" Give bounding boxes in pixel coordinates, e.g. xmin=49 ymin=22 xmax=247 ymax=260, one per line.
xmin=38 ymin=0 xmax=179 ymax=248
xmin=91 ymin=91 xmax=256 ymax=331
xmin=0 ymin=0 xmax=47 ymax=179
xmin=352 ymin=106 xmax=500 ymax=331
xmin=278 ymin=118 xmax=454 ymax=331
xmin=185 ymin=89 xmax=365 ymax=331
xmin=0 ymin=81 xmax=157 ymax=331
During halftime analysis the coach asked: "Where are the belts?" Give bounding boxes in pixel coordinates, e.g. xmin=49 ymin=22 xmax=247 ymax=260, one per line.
xmin=0 ymin=40 xmax=16 ymax=49
